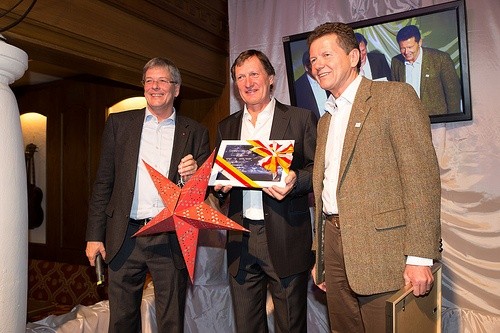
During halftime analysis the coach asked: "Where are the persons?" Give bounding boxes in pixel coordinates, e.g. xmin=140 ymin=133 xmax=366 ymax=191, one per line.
xmin=295 ymin=51 xmax=331 ymax=117
xmin=307 ymin=22 xmax=443 ymax=333
xmin=207 ymin=50 xmax=317 ymax=333
xmin=354 ymin=32 xmax=392 ymax=81
xmin=86 ymin=56 xmax=210 ymax=333
xmin=391 ymin=25 xmax=461 ymax=115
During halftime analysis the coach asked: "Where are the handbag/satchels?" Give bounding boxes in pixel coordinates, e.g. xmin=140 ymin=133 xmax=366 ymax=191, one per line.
xmin=26 ymin=153 xmax=44 ymax=230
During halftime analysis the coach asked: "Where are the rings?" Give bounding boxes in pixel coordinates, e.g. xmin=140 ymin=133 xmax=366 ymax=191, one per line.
xmin=426 ymin=290 xmax=430 ymax=292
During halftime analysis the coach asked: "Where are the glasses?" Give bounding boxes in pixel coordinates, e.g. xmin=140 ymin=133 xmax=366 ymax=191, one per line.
xmin=141 ymin=79 xmax=177 ymax=85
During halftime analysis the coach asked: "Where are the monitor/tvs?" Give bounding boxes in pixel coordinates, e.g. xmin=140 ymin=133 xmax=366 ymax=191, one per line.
xmin=282 ymin=1 xmax=473 ymax=125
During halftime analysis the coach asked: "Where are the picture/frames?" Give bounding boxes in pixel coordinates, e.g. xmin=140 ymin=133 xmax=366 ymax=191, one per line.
xmin=207 ymin=139 xmax=295 ymax=188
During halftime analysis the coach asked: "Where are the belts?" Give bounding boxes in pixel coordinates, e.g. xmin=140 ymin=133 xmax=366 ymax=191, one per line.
xmin=325 ymin=214 xmax=340 ymax=229
xmin=243 ymin=219 xmax=265 ymax=225
xmin=129 ymin=218 xmax=150 ymax=226
